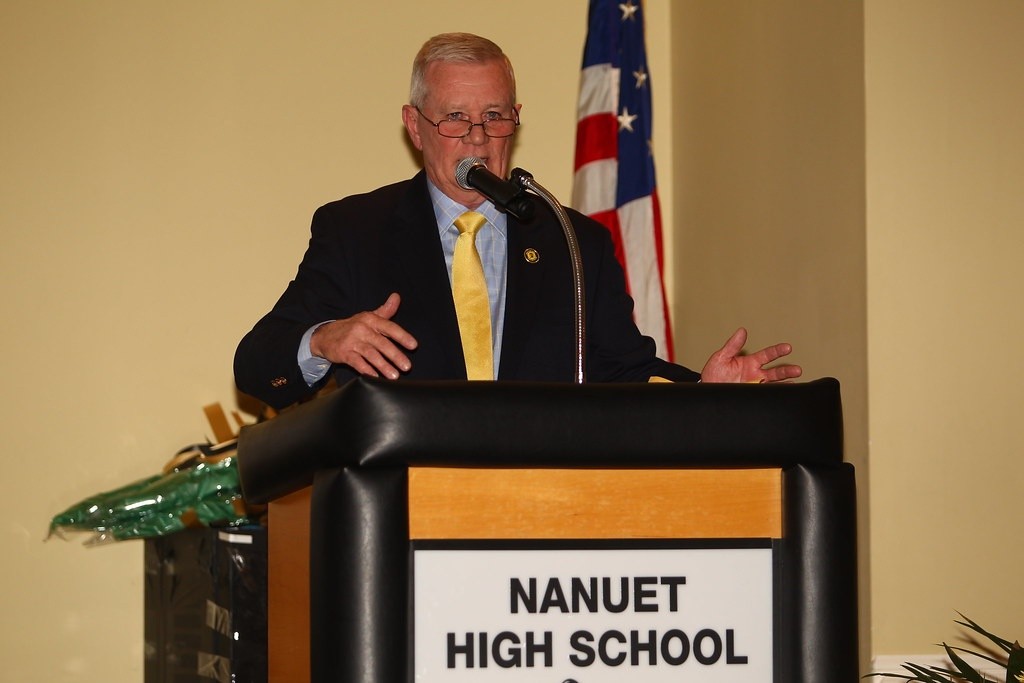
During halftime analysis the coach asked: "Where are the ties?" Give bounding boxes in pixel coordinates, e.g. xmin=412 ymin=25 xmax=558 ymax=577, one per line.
xmin=451 ymin=210 xmax=494 ymax=381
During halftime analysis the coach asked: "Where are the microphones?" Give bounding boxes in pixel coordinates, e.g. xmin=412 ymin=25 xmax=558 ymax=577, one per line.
xmin=453 ymin=156 xmax=545 ymax=224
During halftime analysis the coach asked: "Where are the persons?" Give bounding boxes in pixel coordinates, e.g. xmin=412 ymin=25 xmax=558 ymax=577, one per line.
xmin=232 ymin=32 xmax=803 ymax=414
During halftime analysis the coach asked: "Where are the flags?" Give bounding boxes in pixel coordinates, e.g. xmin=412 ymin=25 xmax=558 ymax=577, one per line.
xmin=570 ymin=0 xmax=677 ymax=365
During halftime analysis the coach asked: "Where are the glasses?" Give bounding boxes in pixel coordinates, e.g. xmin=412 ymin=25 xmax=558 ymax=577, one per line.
xmin=414 ymin=105 xmax=521 ymax=138
